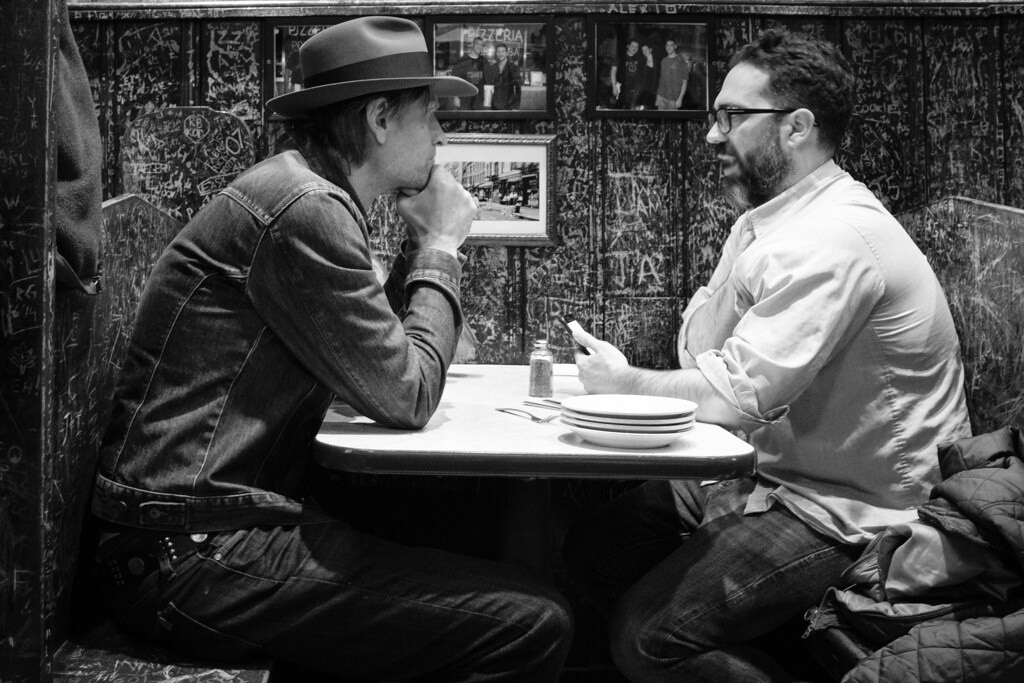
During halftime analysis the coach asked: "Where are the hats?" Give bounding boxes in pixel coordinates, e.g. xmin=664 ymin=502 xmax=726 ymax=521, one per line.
xmin=265 ymin=16 xmax=479 ymax=118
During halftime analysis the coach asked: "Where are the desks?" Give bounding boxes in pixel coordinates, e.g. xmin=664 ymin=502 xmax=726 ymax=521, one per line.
xmin=314 ymin=364 xmax=756 ymax=584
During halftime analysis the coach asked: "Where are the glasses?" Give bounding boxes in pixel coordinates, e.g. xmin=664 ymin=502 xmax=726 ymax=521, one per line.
xmin=706 ymin=108 xmax=819 ymax=134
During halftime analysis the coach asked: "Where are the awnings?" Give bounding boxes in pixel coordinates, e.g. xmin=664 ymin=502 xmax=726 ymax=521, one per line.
xmin=478 ymin=173 xmax=538 ymax=188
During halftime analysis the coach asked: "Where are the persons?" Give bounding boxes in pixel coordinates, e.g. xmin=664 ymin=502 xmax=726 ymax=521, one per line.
xmin=572 ymin=24 xmax=976 ymax=683
xmin=96 ymin=16 xmax=575 ymax=683
xmin=452 ymin=36 xmax=523 ymax=111
xmin=610 ymin=38 xmax=689 ymax=110
xmin=469 ymin=189 xmax=523 ymax=220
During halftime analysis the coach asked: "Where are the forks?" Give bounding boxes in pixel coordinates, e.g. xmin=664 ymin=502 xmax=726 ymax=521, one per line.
xmin=495 ymin=407 xmax=559 ymax=424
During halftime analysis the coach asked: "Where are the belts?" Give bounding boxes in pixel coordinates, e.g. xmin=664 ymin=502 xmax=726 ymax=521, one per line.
xmin=95 ymin=532 xmax=232 ymax=588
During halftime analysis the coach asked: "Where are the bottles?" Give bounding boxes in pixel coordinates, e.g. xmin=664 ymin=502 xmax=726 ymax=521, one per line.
xmin=529 ymin=340 xmax=554 ymax=398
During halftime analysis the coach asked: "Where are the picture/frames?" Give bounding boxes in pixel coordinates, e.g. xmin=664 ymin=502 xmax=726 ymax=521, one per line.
xmin=422 ymin=14 xmax=557 ymax=121
xmin=585 ymin=14 xmax=715 ymax=121
xmin=263 ymin=16 xmax=357 ymax=123
xmin=433 ymin=133 xmax=559 ymax=247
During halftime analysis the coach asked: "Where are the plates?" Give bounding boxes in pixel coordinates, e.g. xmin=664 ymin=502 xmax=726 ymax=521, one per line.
xmin=561 ymin=393 xmax=699 ymax=449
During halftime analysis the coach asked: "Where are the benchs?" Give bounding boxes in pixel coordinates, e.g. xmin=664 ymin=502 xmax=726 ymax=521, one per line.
xmin=809 ymin=192 xmax=1024 ymax=683
xmin=0 ymin=192 xmax=277 ymax=683
